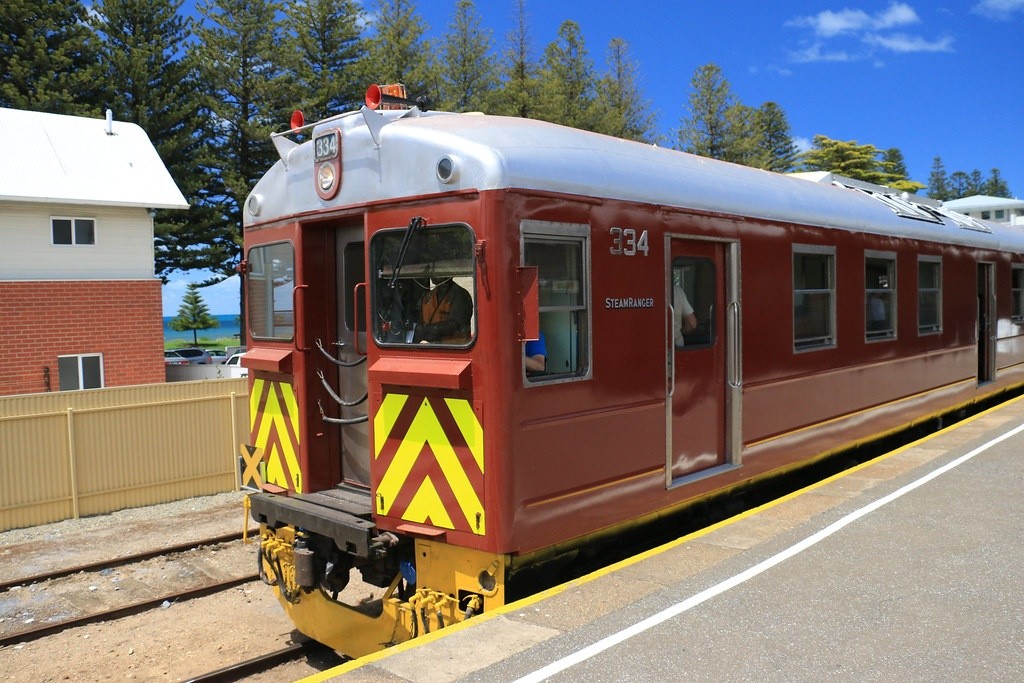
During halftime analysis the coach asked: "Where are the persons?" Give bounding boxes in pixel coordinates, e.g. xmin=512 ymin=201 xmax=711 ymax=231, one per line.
xmin=674 ymin=275 xmax=698 ymax=349
xmin=525 ymin=328 xmax=548 ymax=377
xmin=412 ymin=257 xmax=473 ymax=344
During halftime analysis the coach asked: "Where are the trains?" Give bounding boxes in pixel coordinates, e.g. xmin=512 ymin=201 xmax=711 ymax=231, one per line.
xmin=235 ymin=84 xmax=1024 ymax=660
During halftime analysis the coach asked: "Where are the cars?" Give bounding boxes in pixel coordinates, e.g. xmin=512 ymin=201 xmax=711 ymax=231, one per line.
xmin=164 ymin=348 xmax=248 ymax=378
